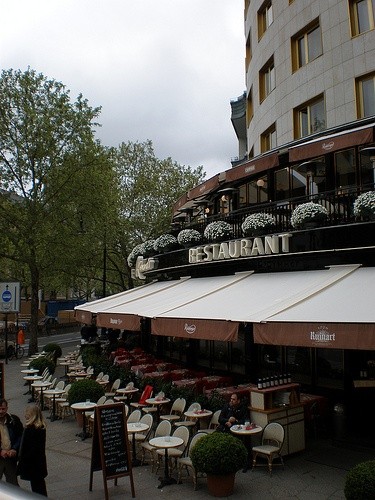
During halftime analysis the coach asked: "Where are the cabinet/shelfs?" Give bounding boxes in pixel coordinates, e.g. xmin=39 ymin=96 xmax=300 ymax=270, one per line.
xmin=247 ymin=382 xmax=311 ymax=465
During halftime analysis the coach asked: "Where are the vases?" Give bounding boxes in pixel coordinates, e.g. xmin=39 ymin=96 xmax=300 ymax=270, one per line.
xmin=163 ymin=244 xmax=171 ymax=253
xmin=149 ymin=249 xmax=156 ymax=257
xmin=367 ymin=211 xmax=375 ymax=221
xmin=303 ymin=215 xmax=319 ymax=229
xmin=214 ymin=235 xmax=222 ymax=243
xmin=182 ymin=241 xmax=193 ymax=249
xmin=253 ymin=225 xmax=266 ymax=236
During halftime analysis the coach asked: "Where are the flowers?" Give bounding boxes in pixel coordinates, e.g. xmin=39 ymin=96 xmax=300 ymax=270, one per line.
xmin=290 ymin=201 xmax=327 ymax=229
xmin=241 ymin=212 xmax=276 ymax=234
xmin=204 ymin=221 xmax=233 ymax=241
xmin=352 ymin=190 xmax=375 ymax=217
xmin=127 ymin=233 xmax=177 ymax=267
xmin=177 ymin=229 xmax=202 ymax=244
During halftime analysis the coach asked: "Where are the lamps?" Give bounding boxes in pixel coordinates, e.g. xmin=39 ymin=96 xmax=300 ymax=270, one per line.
xmin=257 ymin=179 xmax=267 ymax=194
xmin=221 ymin=195 xmax=228 ymax=204
xmin=205 ymin=208 xmax=210 ymax=216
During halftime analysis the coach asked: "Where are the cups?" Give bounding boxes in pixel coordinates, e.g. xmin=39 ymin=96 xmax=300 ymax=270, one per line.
xmin=86 ymin=399 xmax=90 ymax=404
xmin=245 ymin=422 xmax=250 ymax=427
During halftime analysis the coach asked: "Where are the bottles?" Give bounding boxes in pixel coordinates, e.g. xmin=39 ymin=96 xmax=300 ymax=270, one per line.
xmin=257 ymin=373 xmax=292 ymax=389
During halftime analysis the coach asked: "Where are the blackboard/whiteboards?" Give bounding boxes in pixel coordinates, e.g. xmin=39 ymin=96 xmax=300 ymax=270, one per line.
xmin=91 ymin=402 xmax=132 ymax=480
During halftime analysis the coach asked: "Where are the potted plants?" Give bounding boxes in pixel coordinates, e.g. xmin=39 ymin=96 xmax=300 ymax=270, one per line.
xmin=67 ymin=378 xmax=104 ymax=428
xmin=188 ymin=431 xmax=248 ymax=498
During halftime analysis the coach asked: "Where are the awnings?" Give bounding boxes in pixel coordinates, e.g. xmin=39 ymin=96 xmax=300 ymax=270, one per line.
xmin=74 ymin=264 xmax=375 ymax=351
xmin=172 ymin=122 xmax=375 ymax=219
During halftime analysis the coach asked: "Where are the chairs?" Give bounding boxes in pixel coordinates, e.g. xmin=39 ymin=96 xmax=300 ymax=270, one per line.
xmin=25 ymin=348 xmax=285 ymax=491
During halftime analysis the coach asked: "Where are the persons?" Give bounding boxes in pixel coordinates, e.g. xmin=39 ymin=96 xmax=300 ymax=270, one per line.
xmin=17 ymin=405 xmax=48 ymax=497
xmin=214 ymin=393 xmax=246 ymax=435
xmin=0 ymin=397 xmax=23 ymax=486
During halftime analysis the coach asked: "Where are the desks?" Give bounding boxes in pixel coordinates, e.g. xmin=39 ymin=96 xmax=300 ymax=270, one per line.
xmin=20 ymin=369 xmax=40 ymax=395
xmin=148 ymin=436 xmax=185 ymax=489
xmin=30 ymin=382 xmax=53 ymax=411
xmin=184 ymin=409 xmax=213 ymax=445
xmin=22 ymin=375 xmax=44 ymax=403
xmin=70 ymin=402 xmax=97 ymax=441
xmin=230 ymin=424 xmax=263 ymax=473
xmin=126 ymin=422 xmax=150 ymax=468
xmin=42 ymin=389 xmax=66 ymax=422
